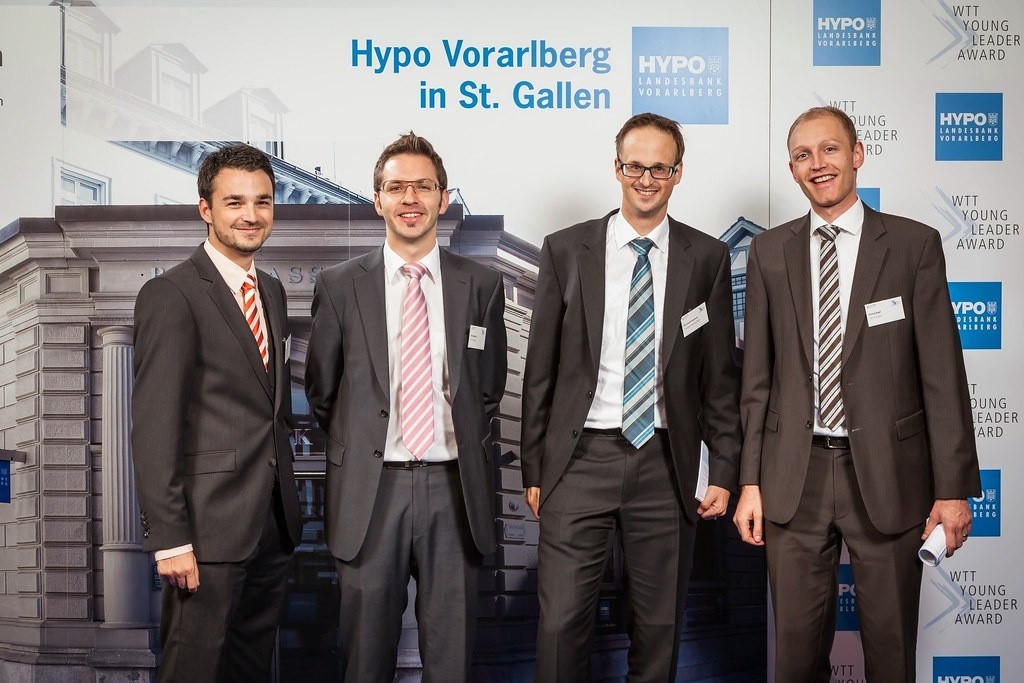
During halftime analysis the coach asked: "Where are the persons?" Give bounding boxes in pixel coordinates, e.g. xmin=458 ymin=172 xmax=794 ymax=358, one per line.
xmin=734 ymin=106 xmax=983 ymax=683
xmin=520 ymin=112 xmax=737 ymax=683
xmin=303 ymin=132 xmax=508 ymax=683
xmin=130 ymin=148 xmax=304 ymax=683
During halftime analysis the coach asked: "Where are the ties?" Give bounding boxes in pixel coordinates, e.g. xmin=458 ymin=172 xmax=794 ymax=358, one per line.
xmin=622 ymin=238 xmax=655 ymax=448
xmin=816 ymin=224 xmax=846 ymax=432
xmin=400 ymin=262 xmax=434 ymax=461
xmin=240 ymin=274 xmax=267 ymax=372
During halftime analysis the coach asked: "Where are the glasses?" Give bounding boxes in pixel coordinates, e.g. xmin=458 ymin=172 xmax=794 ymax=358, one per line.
xmin=377 ymin=179 xmax=443 ymax=194
xmin=617 ymin=158 xmax=681 ymax=179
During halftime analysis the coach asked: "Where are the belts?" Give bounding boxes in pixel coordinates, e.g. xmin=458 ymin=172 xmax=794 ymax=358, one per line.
xmin=811 ymin=435 xmax=850 ymax=449
xmin=383 ymin=460 xmax=459 ymax=468
